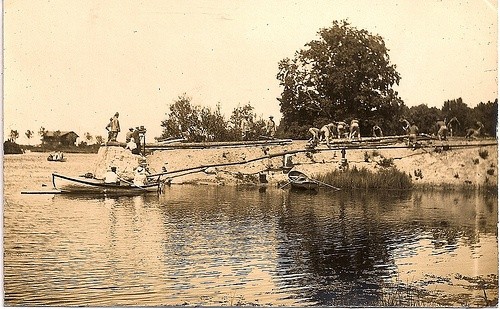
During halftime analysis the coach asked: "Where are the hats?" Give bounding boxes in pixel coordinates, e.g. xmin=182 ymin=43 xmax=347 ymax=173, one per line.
xmin=129 ymin=128 xmax=134 ymax=130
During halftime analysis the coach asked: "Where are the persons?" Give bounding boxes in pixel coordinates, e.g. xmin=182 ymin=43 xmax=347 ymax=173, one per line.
xmin=106 ymin=111 xmax=121 ymax=142
xmin=241 ymin=115 xmax=255 ymax=141
xmin=134 ymin=168 xmax=149 ymax=187
xmin=51 ymin=150 xmax=64 ymax=161
xmin=265 ymin=115 xmax=277 ymax=140
xmin=465 ymin=117 xmax=491 ymax=138
xmin=434 ymin=116 xmax=461 ymax=141
xmin=103 ymin=167 xmax=120 ymax=186
xmin=125 ymin=125 xmax=143 ymax=156
xmin=309 ymin=117 xmax=362 ymax=148
xmin=398 ymin=116 xmax=419 ymax=150
xmin=372 ymin=125 xmax=382 ymax=138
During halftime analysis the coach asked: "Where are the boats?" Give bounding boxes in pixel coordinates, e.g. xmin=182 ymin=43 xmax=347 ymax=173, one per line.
xmin=287 ymin=165 xmax=319 ymax=191
xmin=52 ymin=143 xmax=101 ymax=155
xmin=47 ymin=155 xmax=68 ymax=162
xmin=51 ymin=172 xmax=166 ymax=195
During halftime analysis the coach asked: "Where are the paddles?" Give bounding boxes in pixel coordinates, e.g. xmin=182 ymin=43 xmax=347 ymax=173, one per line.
xmin=121 ymin=178 xmax=145 ymax=192
xmin=311 ymin=177 xmax=341 ymax=191
xmin=280 ymin=175 xmax=300 ymax=190
